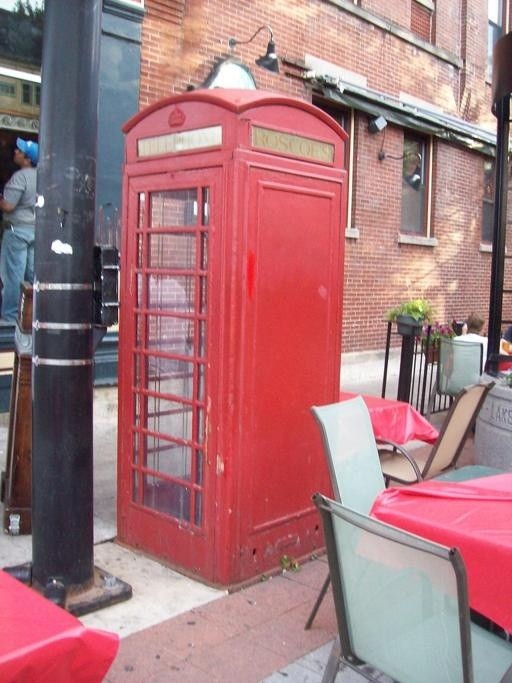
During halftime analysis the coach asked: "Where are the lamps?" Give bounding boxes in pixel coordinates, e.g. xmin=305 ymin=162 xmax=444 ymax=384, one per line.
xmin=229 ymin=24 xmax=280 ymax=75
xmin=378 ymin=151 xmax=422 ymax=192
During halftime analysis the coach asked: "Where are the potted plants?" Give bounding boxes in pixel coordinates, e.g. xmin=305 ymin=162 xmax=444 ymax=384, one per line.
xmin=386 ymin=299 xmax=429 ymax=336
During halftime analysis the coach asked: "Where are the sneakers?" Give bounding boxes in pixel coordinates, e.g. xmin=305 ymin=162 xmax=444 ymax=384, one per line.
xmin=0 ymin=318 xmax=17 ymax=329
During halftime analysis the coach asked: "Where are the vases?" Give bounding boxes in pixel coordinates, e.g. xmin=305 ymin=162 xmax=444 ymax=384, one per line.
xmin=423 ymin=348 xmax=437 ymax=363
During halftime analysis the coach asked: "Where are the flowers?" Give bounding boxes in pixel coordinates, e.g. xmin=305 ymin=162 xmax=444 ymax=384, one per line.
xmin=422 ymin=322 xmax=453 ymax=349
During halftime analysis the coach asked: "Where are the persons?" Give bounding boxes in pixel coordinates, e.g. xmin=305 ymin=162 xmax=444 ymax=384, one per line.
xmin=498 ymin=325 xmax=512 ymax=372
xmin=0 ymin=136 xmax=39 ymax=326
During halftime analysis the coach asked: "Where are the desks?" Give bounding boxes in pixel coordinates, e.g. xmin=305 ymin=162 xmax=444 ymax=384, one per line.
xmin=340 ymin=392 xmax=440 ymax=452
xmin=370 ymin=463 xmax=512 ymax=636
xmin=0 ymin=565 xmax=120 ymax=683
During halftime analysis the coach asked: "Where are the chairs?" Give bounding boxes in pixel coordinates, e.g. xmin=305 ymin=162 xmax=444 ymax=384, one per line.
xmin=304 ymin=395 xmax=388 ymax=629
xmin=436 ymin=337 xmax=483 ymax=433
xmin=312 ymin=493 xmax=512 ymax=683
xmin=375 ymin=382 xmax=496 ymax=489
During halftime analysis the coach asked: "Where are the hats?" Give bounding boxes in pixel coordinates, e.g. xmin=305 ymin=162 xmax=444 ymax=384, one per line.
xmin=16 ymin=137 xmax=39 ymax=165
